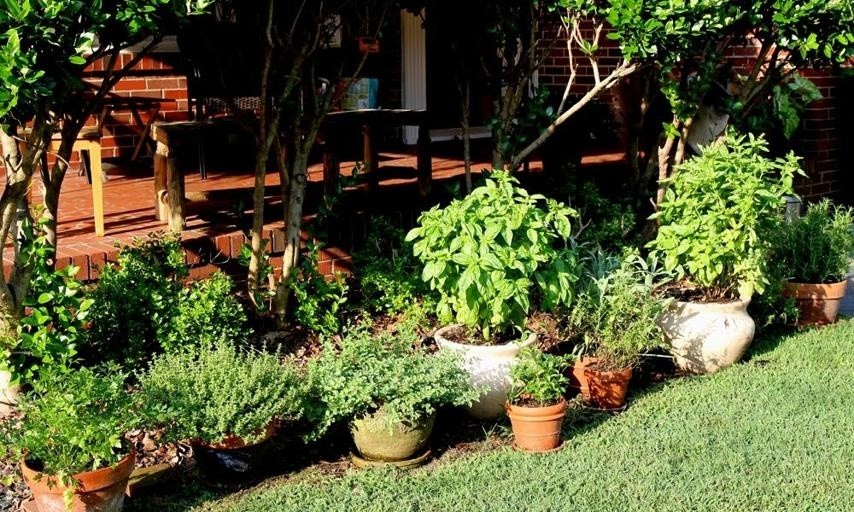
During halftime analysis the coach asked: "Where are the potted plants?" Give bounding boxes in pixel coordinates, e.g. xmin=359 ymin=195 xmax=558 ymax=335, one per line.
xmin=773 ymin=193 xmax=853 ymax=331
xmin=635 ymin=132 xmax=804 ymax=370
xmin=148 ymin=331 xmax=304 ymax=490
xmin=572 ymin=255 xmax=663 ymax=413
xmin=408 ymin=160 xmax=580 ymax=418
xmin=0 ymin=358 xmax=154 ymax=511
xmin=306 ymin=317 xmax=475 ymax=472
xmin=504 ymin=340 xmax=568 ymax=454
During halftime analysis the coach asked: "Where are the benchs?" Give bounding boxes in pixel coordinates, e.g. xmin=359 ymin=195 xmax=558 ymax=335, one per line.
xmin=148 ymin=105 xmax=433 ymax=231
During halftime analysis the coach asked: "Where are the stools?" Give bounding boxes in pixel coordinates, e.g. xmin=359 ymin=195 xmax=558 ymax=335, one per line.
xmin=8 ymin=127 xmax=111 ymax=241
